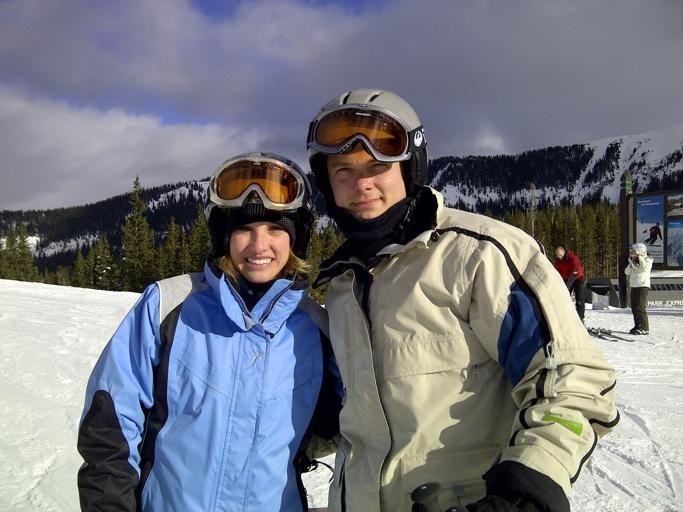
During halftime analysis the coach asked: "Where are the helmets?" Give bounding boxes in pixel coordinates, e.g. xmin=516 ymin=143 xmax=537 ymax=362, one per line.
xmin=308 ymin=88 xmax=429 ymax=198
xmin=203 ymin=152 xmax=313 ymax=258
xmin=629 ymin=242 xmax=647 ymax=259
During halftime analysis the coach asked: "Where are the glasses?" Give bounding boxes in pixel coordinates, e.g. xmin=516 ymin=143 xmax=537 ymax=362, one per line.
xmin=306 ymin=103 xmax=427 ymax=162
xmin=209 ymin=156 xmax=312 ymax=213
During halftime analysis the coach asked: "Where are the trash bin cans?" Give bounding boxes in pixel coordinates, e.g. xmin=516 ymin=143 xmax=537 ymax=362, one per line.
xmin=590 ymin=286 xmax=611 ymax=310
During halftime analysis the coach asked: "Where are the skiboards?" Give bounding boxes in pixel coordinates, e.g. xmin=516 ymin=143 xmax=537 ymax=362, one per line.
xmin=586 ymin=327 xmax=635 ymax=342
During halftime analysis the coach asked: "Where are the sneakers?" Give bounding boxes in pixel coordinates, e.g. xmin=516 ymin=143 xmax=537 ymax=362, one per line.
xmin=630 ymin=326 xmax=649 ymax=334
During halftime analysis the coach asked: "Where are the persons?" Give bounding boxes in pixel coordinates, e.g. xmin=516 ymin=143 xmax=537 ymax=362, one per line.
xmin=306 ymin=90 xmax=621 ymax=512
xmin=623 ymin=243 xmax=654 ymax=336
xmin=552 ymin=245 xmax=587 ymax=329
xmin=76 ymin=151 xmax=344 ymax=512
xmin=645 ymin=222 xmax=662 ymax=245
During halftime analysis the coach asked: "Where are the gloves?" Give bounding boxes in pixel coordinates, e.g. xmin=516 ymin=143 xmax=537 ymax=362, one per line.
xmin=444 ymin=460 xmax=570 ymax=512
